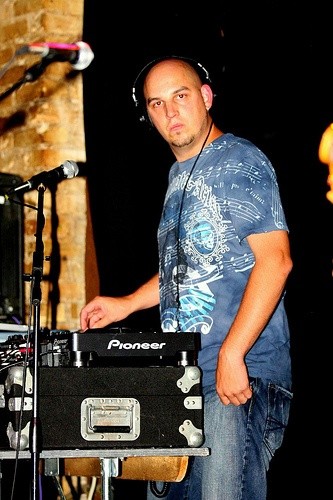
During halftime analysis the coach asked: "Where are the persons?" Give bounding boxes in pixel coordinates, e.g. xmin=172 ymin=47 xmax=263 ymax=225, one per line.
xmin=79 ymin=59 xmax=293 ymax=500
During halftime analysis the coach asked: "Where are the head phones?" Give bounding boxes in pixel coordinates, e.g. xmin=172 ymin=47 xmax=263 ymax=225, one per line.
xmin=130 ymin=53 xmax=218 ymax=130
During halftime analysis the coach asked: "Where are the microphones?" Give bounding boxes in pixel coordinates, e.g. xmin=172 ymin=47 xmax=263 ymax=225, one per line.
xmin=15 ymin=38 xmax=96 ymax=72
xmin=4 ymin=159 xmax=80 ymax=195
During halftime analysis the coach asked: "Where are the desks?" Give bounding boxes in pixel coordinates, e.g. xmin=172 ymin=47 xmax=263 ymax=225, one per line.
xmin=0 ymin=445 xmax=213 ymax=500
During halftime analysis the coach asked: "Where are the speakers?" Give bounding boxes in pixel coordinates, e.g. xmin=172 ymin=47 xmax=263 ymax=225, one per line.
xmin=0 ymin=173 xmax=25 ymax=324
